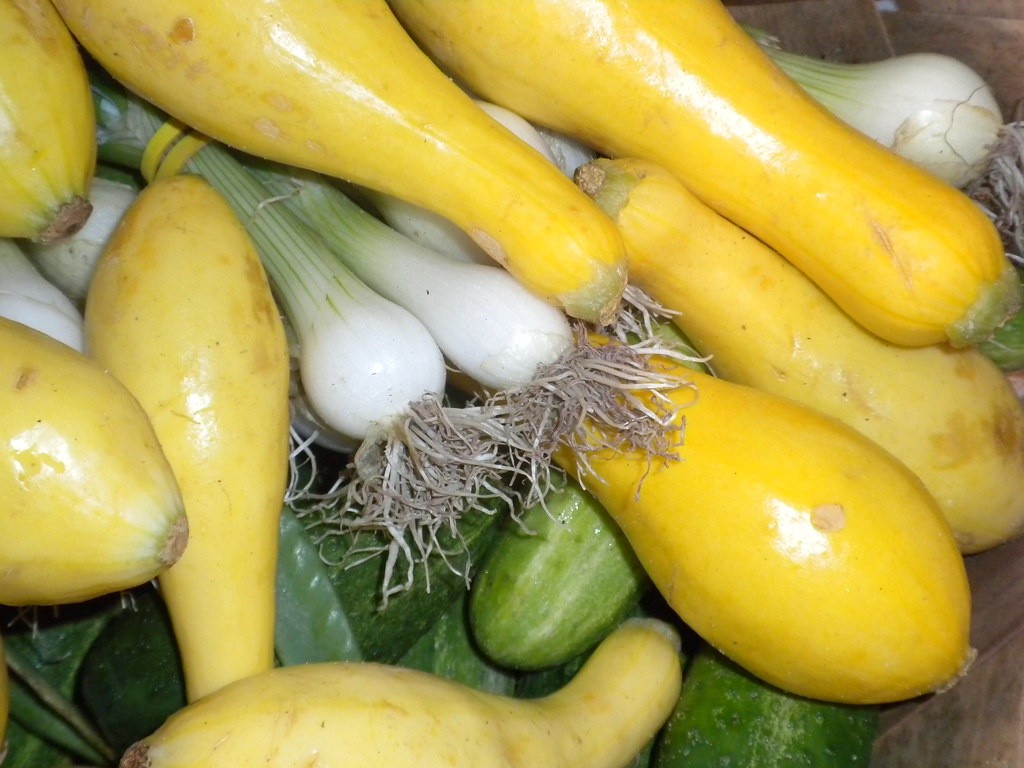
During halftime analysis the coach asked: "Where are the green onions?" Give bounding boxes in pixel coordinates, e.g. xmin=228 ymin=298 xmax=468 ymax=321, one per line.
xmin=1 ymin=34 xmax=1024 ymax=610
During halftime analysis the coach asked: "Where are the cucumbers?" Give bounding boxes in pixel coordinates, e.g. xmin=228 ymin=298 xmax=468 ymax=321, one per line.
xmin=81 ymin=283 xmax=1023 ymax=768
